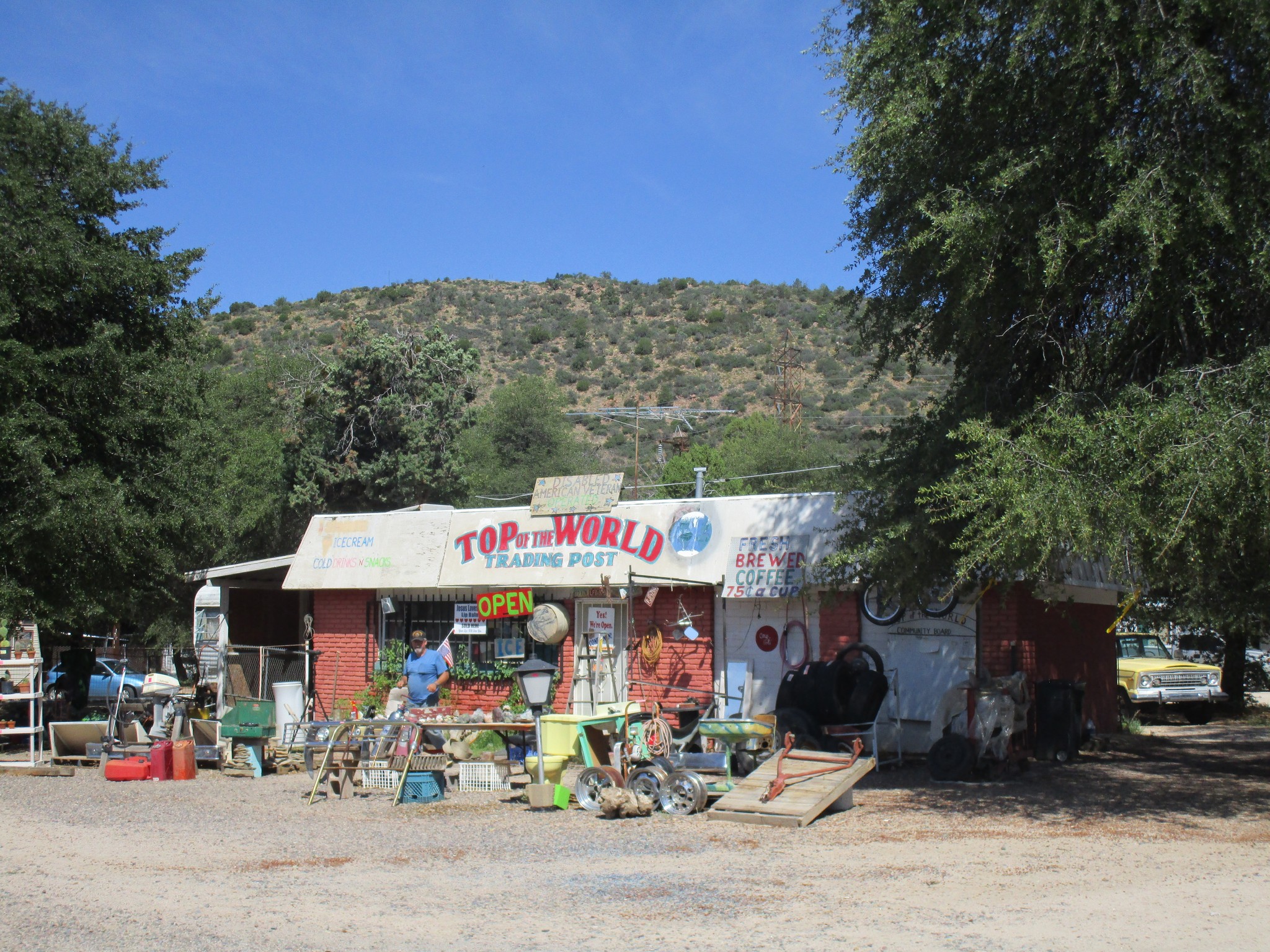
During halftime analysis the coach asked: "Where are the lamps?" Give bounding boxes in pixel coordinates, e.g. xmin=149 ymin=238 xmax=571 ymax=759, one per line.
xmin=513 ymin=651 xmax=560 ymax=812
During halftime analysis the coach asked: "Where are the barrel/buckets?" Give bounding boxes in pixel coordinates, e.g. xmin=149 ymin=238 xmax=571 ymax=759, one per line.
xmin=151 ymin=741 xmax=174 ymax=780
xmin=172 ymin=741 xmax=196 ymax=780
xmin=104 ymin=759 xmax=152 ymax=781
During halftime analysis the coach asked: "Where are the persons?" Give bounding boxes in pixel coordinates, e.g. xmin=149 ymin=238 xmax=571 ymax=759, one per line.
xmin=395 ymin=629 xmax=450 ymax=713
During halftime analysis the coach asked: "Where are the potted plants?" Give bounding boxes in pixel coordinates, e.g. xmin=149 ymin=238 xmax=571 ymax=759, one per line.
xmin=13 ymin=640 xmax=22 ymax=660
xmin=0 ymin=719 xmax=7 ymax=729
xmin=0 ymin=617 xmax=12 ymax=659
xmin=18 ymin=677 xmax=30 ymax=694
xmin=6 ymin=719 xmax=16 ymax=728
xmin=26 ymin=639 xmax=35 ymax=659
xmin=2 ymin=669 xmax=13 ymax=694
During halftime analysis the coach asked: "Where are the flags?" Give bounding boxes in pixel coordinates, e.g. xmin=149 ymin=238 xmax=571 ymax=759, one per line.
xmin=435 ymin=638 xmax=455 ymax=668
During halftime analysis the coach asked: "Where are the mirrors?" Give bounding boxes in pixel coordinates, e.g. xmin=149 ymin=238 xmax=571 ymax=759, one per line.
xmin=711 ymin=653 xmax=754 ymax=754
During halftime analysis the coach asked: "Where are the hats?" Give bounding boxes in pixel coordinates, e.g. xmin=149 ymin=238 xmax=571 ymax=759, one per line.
xmin=411 ymin=630 xmax=426 ymax=642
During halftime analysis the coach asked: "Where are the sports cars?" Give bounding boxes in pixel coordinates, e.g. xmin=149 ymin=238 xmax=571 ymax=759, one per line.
xmin=1115 ymin=632 xmax=1229 ymax=725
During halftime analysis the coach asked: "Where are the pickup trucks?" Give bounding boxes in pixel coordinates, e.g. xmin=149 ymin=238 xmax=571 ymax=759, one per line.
xmin=1143 ymin=626 xmax=1270 ymax=692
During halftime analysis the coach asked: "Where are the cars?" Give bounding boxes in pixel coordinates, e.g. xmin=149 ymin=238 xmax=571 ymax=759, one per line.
xmin=47 ymin=657 xmax=153 ymax=700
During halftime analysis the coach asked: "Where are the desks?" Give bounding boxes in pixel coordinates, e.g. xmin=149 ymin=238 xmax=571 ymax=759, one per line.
xmin=418 ymin=721 xmax=537 ymax=783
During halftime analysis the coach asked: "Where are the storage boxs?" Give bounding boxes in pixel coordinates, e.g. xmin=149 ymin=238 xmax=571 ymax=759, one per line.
xmin=397 ymin=769 xmax=446 ymax=803
xmin=362 ymin=760 xmax=400 ymax=789
xmin=458 ymin=761 xmax=512 ymax=791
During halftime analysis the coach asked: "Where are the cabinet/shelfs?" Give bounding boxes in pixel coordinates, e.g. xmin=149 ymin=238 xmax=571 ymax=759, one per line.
xmin=0 ymin=657 xmax=45 ymax=768
